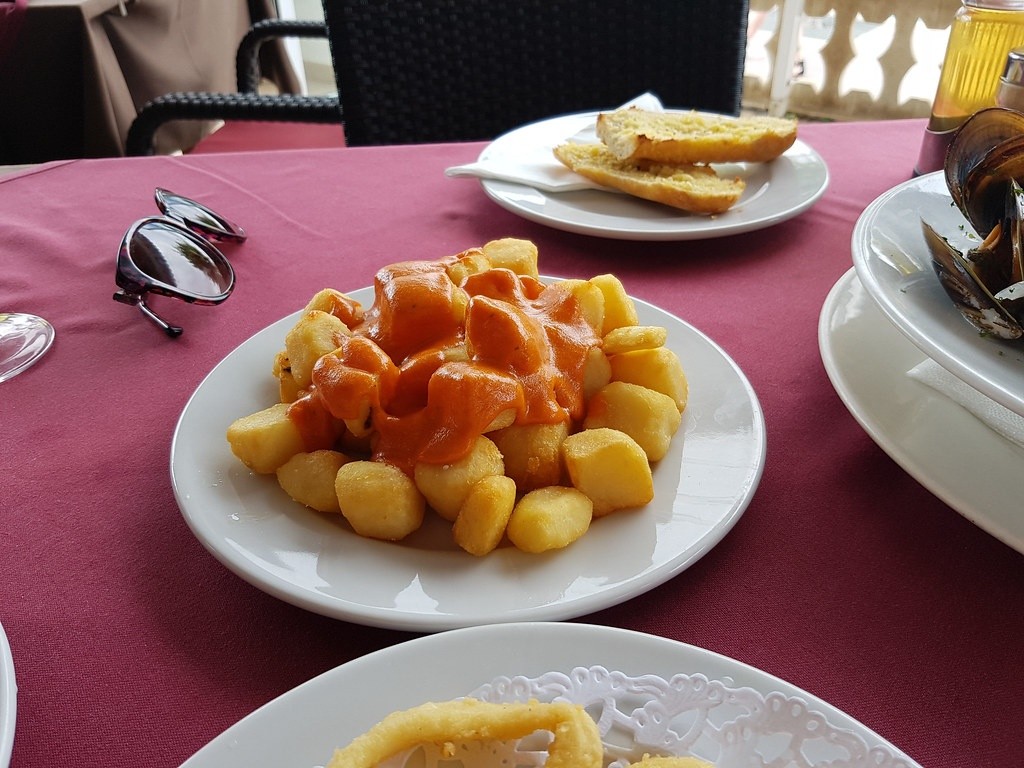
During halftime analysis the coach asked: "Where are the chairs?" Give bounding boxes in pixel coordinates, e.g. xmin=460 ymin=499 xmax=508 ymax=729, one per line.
xmin=124 ymin=0 xmax=751 ymax=157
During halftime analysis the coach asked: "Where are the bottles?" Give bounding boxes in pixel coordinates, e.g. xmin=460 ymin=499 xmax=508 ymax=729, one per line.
xmin=913 ymin=1 xmax=1024 ymax=176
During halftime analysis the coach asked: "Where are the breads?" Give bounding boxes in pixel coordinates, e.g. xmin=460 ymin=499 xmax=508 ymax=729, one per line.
xmin=553 ymin=143 xmax=747 ymax=214
xmin=596 ymin=106 xmax=797 ymax=163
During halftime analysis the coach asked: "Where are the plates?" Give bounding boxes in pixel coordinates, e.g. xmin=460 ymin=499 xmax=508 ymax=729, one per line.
xmin=851 ymin=172 xmax=1023 ymax=417
xmin=0 ymin=621 xmax=17 ymax=768
xmin=168 ymin=274 xmax=768 ymax=632
xmin=476 ymin=109 xmax=830 ymax=240
xmin=178 ymin=622 xmax=924 ymax=768
xmin=818 ymin=266 xmax=1024 ymax=555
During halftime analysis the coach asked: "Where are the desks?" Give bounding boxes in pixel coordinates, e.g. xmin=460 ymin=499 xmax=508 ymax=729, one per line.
xmin=0 ymin=115 xmax=1023 ymax=768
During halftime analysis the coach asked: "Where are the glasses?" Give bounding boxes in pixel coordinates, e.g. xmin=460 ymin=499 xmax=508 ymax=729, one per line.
xmin=112 ymin=188 xmax=246 ymax=337
